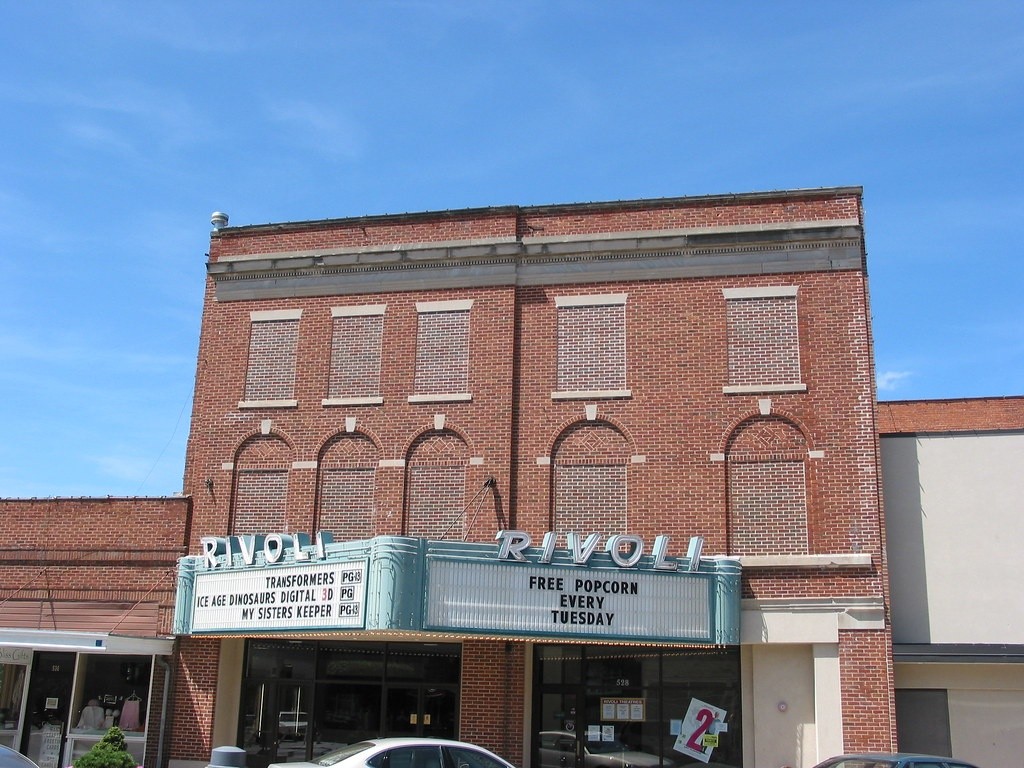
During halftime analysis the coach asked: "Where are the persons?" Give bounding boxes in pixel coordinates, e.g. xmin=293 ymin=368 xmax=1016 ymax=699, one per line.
xmin=620 ymin=721 xmax=632 ymax=745
xmin=396 ymin=708 xmax=409 ymax=731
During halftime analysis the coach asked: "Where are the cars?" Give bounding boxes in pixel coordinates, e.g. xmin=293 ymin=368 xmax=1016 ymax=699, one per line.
xmin=538 ymin=729 xmax=677 ymax=768
xmin=278 ymin=710 xmax=318 ymax=740
xmin=267 ymin=736 xmax=518 ymax=768
xmin=0 ymin=744 xmax=41 ymax=768
xmin=779 ymin=752 xmax=977 ymax=768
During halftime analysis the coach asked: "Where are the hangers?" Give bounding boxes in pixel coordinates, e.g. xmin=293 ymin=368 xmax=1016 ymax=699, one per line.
xmin=126 ymin=691 xmax=143 ymax=703
